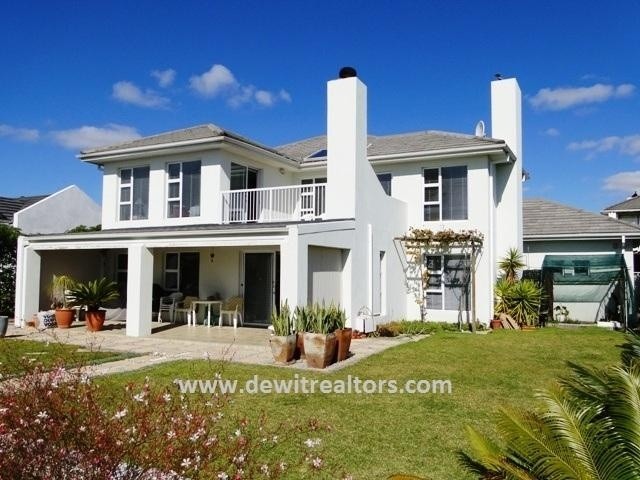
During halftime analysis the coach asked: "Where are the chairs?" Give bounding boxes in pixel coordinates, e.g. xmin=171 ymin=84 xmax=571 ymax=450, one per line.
xmin=158 ymin=291 xmax=243 ymax=329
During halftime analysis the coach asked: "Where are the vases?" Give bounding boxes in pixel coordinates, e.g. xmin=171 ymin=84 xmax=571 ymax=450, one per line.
xmin=43 ymin=313 xmax=56 ymax=329
xmin=0 ymin=315 xmax=9 ymax=337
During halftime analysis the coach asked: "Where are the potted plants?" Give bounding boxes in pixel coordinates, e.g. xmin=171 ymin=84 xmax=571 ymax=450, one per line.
xmin=269 ymin=295 xmax=352 ymax=368
xmin=490 ymin=247 xmax=548 ymax=329
xmin=51 ymin=272 xmax=120 ymax=331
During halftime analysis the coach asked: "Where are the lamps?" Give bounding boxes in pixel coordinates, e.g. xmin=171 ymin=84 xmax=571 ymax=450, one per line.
xmin=279 ymin=167 xmax=285 ymax=177
xmin=208 ymin=247 xmax=215 ymax=264
